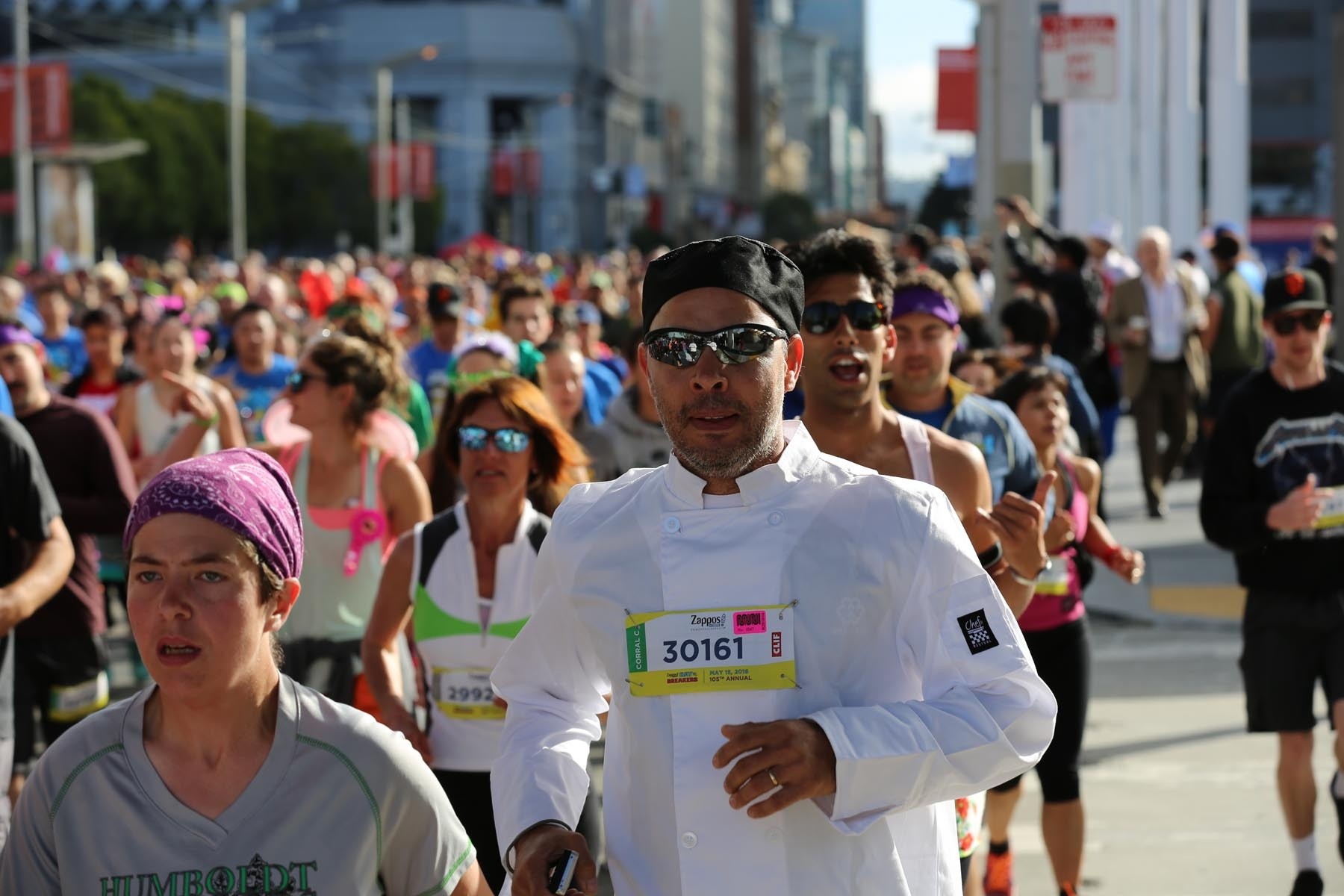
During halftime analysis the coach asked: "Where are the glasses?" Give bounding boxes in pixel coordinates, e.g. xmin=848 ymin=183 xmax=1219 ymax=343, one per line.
xmin=458 ymin=425 xmax=535 ymax=453
xmin=1271 ymin=309 xmax=1325 ymax=334
xmin=646 ymin=323 xmax=789 ymax=369
xmin=289 ymin=371 xmax=325 ymax=392
xmin=802 ymin=298 xmax=885 ymax=335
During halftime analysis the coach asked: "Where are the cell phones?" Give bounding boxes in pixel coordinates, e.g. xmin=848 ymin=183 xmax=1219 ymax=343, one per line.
xmin=548 ymin=848 xmax=580 ymax=896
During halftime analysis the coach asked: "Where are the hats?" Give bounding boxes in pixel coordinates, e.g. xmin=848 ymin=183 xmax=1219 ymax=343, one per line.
xmin=641 ymin=235 xmax=806 ymax=340
xmin=1263 ymin=271 xmax=1324 ymax=315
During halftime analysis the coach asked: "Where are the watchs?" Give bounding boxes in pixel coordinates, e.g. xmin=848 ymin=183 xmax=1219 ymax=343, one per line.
xmin=1009 ymin=553 xmax=1052 ymax=586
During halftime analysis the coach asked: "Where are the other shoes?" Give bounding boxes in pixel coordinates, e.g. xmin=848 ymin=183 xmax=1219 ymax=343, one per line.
xmin=1148 ymin=500 xmax=1162 ymax=517
xmin=982 ymin=846 xmax=1011 ymax=896
xmin=1330 ymin=775 xmax=1344 ymax=860
xmin=1288 ymin=869 xmax=1324 ymax=896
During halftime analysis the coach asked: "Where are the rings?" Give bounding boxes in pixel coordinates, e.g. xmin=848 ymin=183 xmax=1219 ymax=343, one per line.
xmin=768 ymin=769 xmax=778 ymax=786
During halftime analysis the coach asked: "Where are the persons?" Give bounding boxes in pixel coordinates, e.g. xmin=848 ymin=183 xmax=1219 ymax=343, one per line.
xmin=1 ymin=194 xmax=1344 ymax=896
